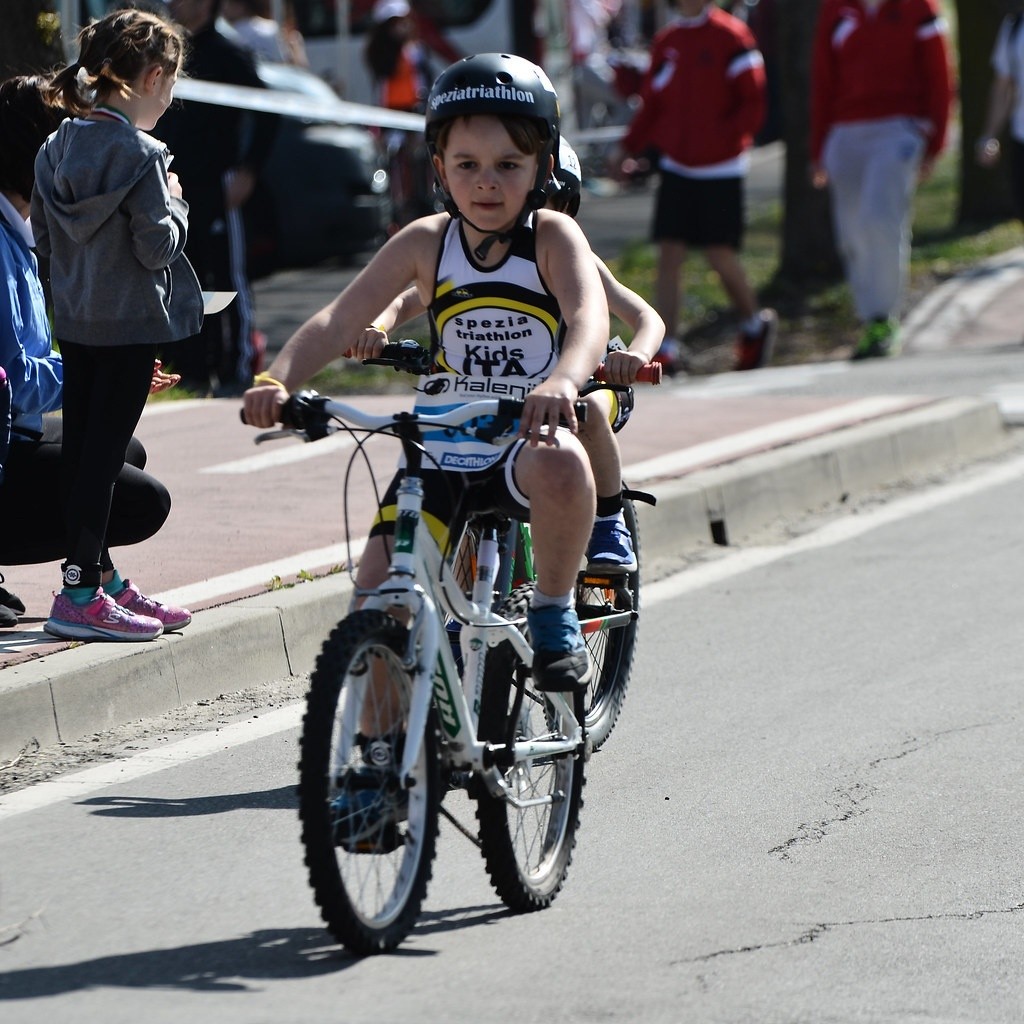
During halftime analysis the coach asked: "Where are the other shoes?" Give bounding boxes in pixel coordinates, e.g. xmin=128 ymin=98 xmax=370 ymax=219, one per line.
xmin=0 ymin=575 xmax=26 ymax=626
xmin=214 ymin=384 xmax=246 ymax=398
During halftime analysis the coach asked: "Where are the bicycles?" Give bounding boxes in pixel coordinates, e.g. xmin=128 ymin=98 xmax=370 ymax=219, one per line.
xmin=342 ymin=338 xmax=662 ymax=792
xmin=239 ymin=389 xmax=588 ymax=958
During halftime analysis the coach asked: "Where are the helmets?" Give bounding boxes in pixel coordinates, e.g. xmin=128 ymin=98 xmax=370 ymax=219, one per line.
xmin=422 ymin=53 xmax=559 ymax=151
xmin=551 ymin=134 xmax=584 ymax=207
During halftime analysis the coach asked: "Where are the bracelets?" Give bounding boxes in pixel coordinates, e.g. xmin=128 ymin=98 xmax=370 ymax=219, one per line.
xmin=254 ymin=373 xmax=286 ymax=394
xmin=371 ymin=322 xmax=386 ymax=334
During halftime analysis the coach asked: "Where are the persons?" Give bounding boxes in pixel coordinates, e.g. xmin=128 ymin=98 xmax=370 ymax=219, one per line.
xmin=0 ymin=62 xmax=174 ymax=632
xmin=972 ymin=0 xmax=1023 ymax=234
xmin=363 ymin=0 xmax=437 ymax=226
xmin=174 ymin=0 xmax=374 ymax=266
xmin=244 ymin=51 xmax=669 ymax=855
xmin=746 ymin=0 xmax=952 ymax=363
xmin=615 ymin=0 xmax=780 ymax=373
xmin=28 ymin=8 xmax=203 ymax=641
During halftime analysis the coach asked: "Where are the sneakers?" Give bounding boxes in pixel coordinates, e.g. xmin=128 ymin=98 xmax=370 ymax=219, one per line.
xmin=584 ymin=515 xmax=637 ymax=574
xmin=44 ymin=586 xmax=161 ymax=642
xmin=733 ymin=310 xmax=778 ymax=370
xmin=324 ymin=786 xmax=409 ymax=845
xmin=108 ymin=581 xmax=192 ymax=630
xmin=523 ymin=598 xmax=593 ymax=689
xmin=641 ymin=354 xmax=689 ymax=381
xmin=852 ymin=314 xmax=900 ymax=360
